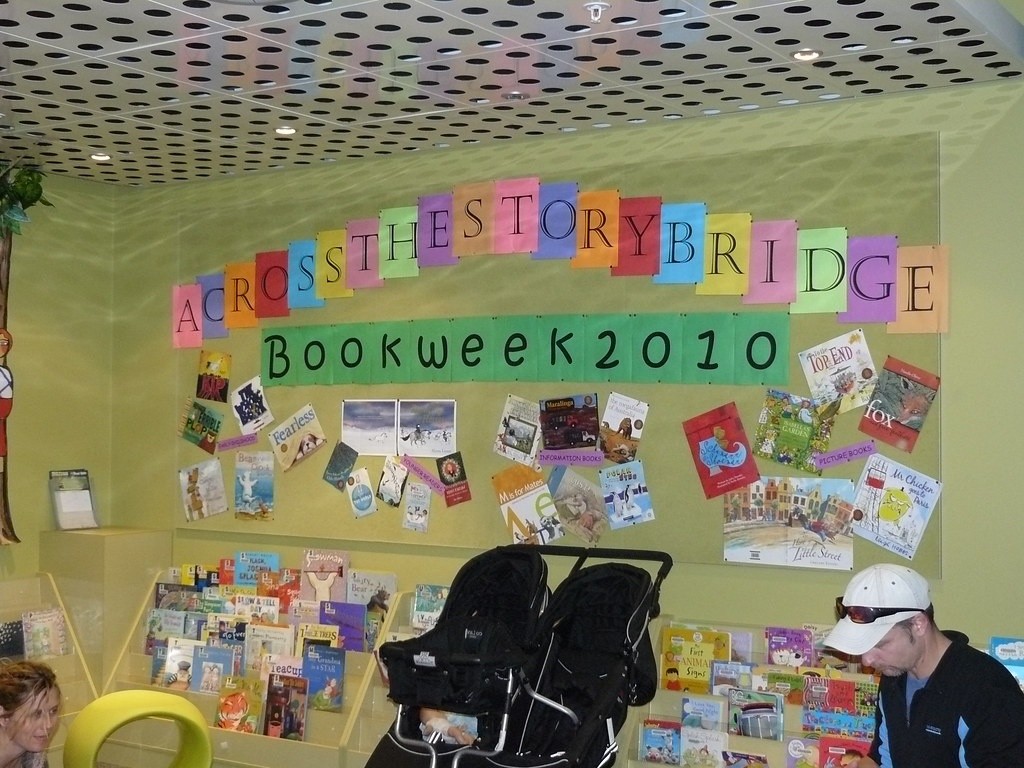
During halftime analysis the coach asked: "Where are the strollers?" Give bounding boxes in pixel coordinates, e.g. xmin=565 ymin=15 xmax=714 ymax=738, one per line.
xmin=365 ymin=543 xmax=673 ymax=768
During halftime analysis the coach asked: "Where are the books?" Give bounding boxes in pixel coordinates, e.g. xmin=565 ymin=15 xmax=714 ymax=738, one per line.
xmin=410 ymin=584 xmax=450 ymax=641
xmin=638 ymin=620 xmax=882 ymax=768
xmin=262 ymin=674 xmax=307 ymax=742
xmin=144 ymin=549 xmax=400 ymax=715
xmin=213 ymin=674 xmax=265 ymax=734
xmin=988 ymin=635 xmax=1023 ymax=692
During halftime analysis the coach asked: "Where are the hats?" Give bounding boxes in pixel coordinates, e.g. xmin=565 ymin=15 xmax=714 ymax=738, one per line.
xmin=822 ymin=562 xmax=932 ymax=655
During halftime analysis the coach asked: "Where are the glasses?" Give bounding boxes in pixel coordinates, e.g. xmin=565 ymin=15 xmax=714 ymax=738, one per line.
xmin=835 ymin=596 xmax=926 ymax=624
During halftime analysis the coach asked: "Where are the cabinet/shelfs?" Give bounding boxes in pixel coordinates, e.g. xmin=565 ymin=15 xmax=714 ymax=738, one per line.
xmin=342 ymin=592 xmax=659 ymax=768
xmin=1 ymin=573 xmax=100 ymax=768
xmin=97 ymin=568 xmax=401 ymax=768
xmin=613 ymin=614 xmax=885 ymax=767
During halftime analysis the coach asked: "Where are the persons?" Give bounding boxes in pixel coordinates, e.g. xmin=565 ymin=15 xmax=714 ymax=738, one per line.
xmin=822 ymin=562 xmax=1024 ymax=768
xmin=0 ymin=661 xmax=59 ymax=768
xmin=419 ymin=707 xmax=475 ymax=746
